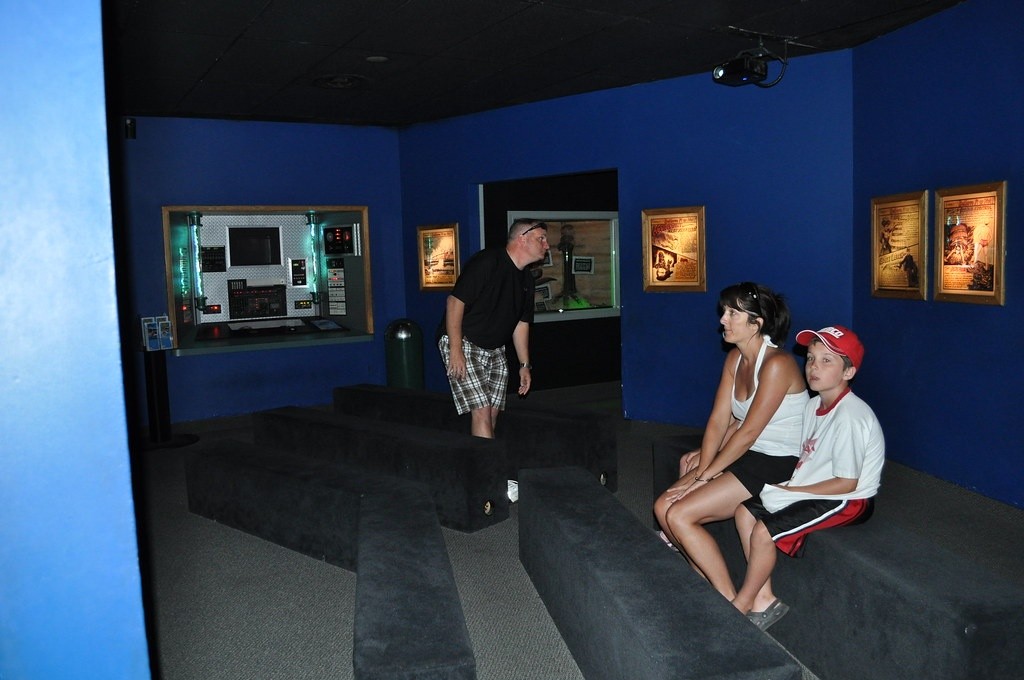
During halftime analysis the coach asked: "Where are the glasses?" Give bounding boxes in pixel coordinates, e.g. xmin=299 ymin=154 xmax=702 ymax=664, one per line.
xmin=522 ymin=223 xmax=548 ymax=235
xmin=741 ymin=282 xmax=763 ymax=310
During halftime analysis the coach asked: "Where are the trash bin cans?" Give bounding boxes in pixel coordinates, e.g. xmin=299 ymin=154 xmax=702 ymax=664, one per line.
xmin=383 ymin=318 xmax=424 ymax=390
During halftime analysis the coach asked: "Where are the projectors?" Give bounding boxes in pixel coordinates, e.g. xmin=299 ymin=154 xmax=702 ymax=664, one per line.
xmin=712 ymin=57 xmax=767 ymax=87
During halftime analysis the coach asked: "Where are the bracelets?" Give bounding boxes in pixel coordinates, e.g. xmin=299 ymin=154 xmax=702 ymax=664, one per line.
xmin=695 ymin=477 xmax=713 ymax=481
xmin=520 ymin=363 xmax=532 ymax=369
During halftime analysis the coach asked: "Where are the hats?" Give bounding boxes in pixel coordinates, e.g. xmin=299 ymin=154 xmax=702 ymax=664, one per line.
xmin=796 ymin=325 xmax=865 ymax=372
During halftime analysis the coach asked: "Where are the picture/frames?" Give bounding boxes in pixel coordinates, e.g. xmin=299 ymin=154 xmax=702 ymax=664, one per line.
xmin=288 ymin=256 xmax=309 ymax=288
xmin=869 ymin=190 xmax=928 ymax=301
xmin=641 ymin=207 xmax=707 ymax=294
xmin=416 ymin=222 xmax=459 ymax=291
xmin=934 ymin=179 xmax=1007 ymax=306
xmin=226 ymin=225 xmax=285 ymax=269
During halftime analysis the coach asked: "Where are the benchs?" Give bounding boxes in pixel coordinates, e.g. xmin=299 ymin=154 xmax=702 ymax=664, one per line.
xmin=517 ymin=467 xmax=821 ymax=680
xmin=183 ymin=438 xmax=402 ymax=572
xmin=331 ymin=384 xmax=621 ymax=494
xmin=254 ymin=405 xmax=511 ymax=531
xmin=351 ymin=480 xmax=476 ymax=679
xmin=651 ymin=430 xmax=1024 ymax=680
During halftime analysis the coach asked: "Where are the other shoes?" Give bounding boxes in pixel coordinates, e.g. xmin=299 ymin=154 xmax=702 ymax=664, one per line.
xmin=505 ymin=480 xmax=520 ymax=504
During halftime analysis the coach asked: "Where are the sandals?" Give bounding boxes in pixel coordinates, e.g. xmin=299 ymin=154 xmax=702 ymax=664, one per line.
xmin=746 ymin=598 xmax=790 ymax=632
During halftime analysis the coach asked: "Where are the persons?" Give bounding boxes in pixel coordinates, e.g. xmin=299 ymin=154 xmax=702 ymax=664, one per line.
xmin=731 ymin=325 xmax=885 ymax=631
xmin=880 ymin=222 xmax=897 ymax=255
xmin=438 ymin=218 xmax=550 ymax=438
xmin=898 ymin=247 xmax=918 ymax=287
xmin=653 ymin=282 xmax=810 ymax=601
